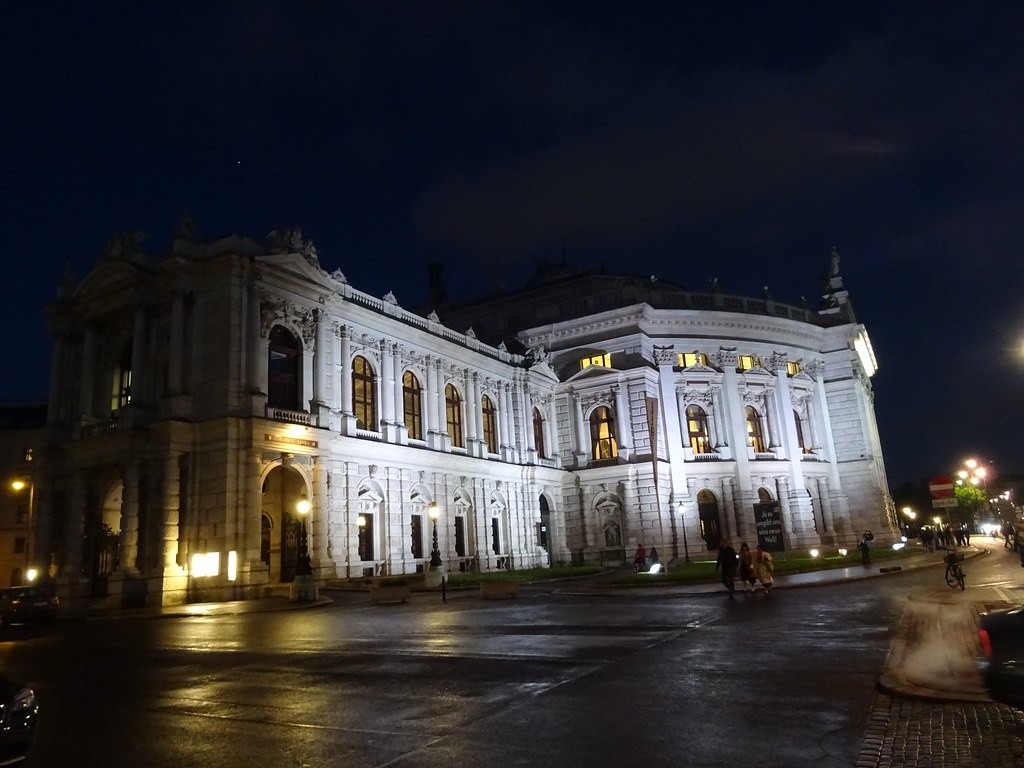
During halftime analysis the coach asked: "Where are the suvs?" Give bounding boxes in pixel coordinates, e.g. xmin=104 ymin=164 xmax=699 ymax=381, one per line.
xmin=0 ymin=584 xmax=62 ymax=631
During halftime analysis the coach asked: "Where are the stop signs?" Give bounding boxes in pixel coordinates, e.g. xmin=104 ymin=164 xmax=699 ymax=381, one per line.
xmin=928 ymin=475 xmax=954 ymax=499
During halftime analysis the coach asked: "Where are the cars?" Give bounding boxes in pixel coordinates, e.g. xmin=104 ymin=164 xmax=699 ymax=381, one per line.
xmin=975 ymin=603 xmax=1024 ymax=712
xmin=0 ymin=674 xmax=40 ymax=768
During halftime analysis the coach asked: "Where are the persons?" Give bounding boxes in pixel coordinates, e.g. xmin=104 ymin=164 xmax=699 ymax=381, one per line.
xmin=268 ymin=225 xmax=323 ymax=269
xmin=634 ymin=544 xmax=658 ymax=572
xmin=999 ymin=520 xmax=1020 ymax=552
xmin=921 ymin=524 xmax=970 ymax=552
xmin=604 ymin=522 xmax=618 ymax=543
xmin=857 ymin=529 xmax=876 ymax=556
xmin=716 ymin=538 xmax=775 ymax=599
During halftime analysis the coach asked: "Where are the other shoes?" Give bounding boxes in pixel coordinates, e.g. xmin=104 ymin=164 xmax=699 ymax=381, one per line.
xmin=744 ymin=587 xmax=751 ymax=593
xmin=751 ymin=587 xmax=756 ymax=592
xmin=765 ymin=589 xmax=768 ymax=594
xmin=728 ymin=589 xmax=735 ymax=594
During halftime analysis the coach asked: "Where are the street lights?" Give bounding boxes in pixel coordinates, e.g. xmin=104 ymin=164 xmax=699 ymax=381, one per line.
xmin=11 ymin=477 xmax=34 ymax=583
xmin=958 ymin=458 xmax=987 ymax=536
xmin=676 ymin=500 xmax=690 ymax=562
xmin=423 ymin=501 xmax=449 ymax=588
xmin=289 ymin=499 xmax=319 ymax=602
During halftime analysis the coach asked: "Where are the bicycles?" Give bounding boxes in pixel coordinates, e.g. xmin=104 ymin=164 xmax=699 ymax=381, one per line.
xmin=942 ymin=546 xmax=966 ymax=591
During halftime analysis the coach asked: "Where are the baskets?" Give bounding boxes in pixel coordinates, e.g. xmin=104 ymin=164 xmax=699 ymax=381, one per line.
xmin=944 ymin=554 xmax=956 ymax=563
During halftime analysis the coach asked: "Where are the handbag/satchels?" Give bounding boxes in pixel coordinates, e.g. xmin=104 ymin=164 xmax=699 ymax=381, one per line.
xmin=729 ymin=566 xmax=737 ymax=578
xmin=766 ymin=562 xmax=774 ymax=572
